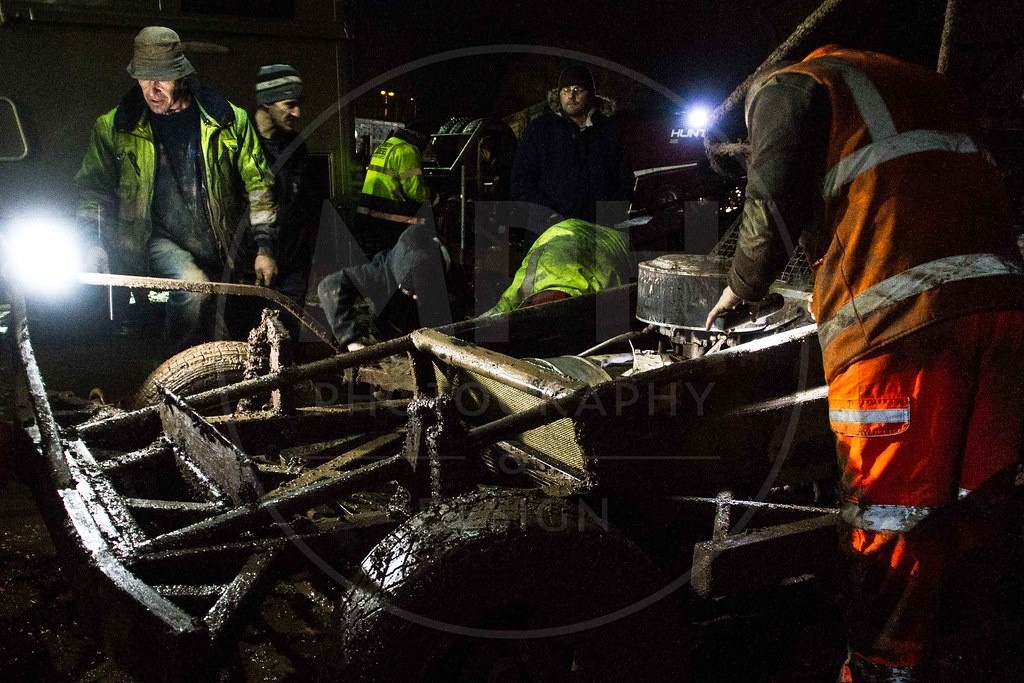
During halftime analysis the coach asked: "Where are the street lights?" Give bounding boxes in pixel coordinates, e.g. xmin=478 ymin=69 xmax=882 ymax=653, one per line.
xmin=411 ymin=98 xmax=417 ymax=117
xmin=381 ymin=90 xmax=395 ymax=118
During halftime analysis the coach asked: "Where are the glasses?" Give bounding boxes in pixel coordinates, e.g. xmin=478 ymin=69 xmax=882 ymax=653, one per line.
xmin=561 ymin=86 xmax=588 ymax=94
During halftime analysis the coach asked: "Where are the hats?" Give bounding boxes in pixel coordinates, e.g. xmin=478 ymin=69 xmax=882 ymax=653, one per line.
xmin=481 ymin=136 xmax=502 ymax=152
xmin=404 ymin=118 xmax=432 ymax=142
xmin=255 ymin=63 xmax=303 ymax=106
xmin=557 ymin=63 xmax=595 ymax=96
xmin=126 ymin=26 xmax=196 ymax=81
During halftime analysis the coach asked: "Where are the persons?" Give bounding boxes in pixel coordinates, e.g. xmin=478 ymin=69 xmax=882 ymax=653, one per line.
xmin=707 ymin=45 xmax=1024 ymax=683
xmin=71 ymin=27 xmax=741 ymax=376
